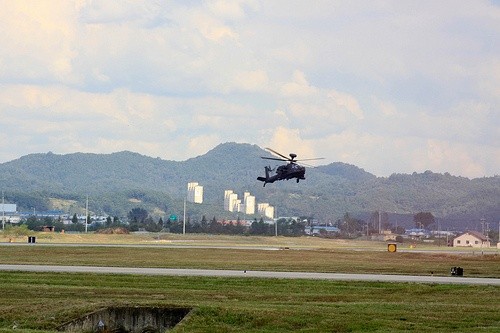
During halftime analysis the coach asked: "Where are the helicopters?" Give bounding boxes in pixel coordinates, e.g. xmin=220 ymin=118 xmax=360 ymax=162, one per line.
xmin=256 ymin=148 xmax=325 ymax=187
